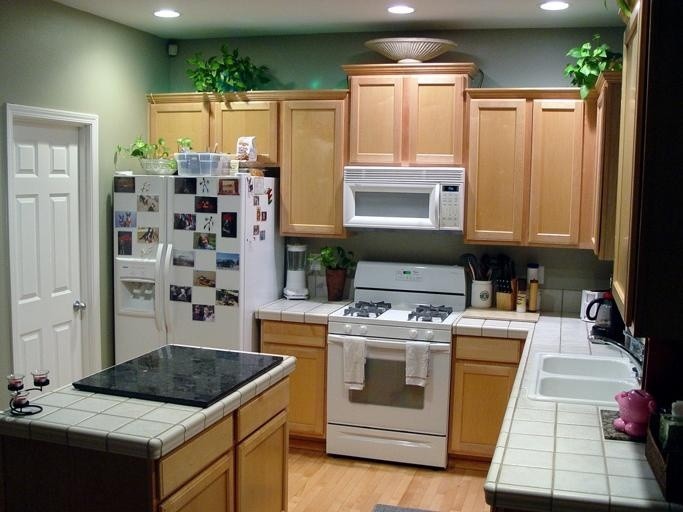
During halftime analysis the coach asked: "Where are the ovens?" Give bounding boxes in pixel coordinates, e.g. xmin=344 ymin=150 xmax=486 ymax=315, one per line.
xmin=326 ymin=336 xmax=451 ymax=470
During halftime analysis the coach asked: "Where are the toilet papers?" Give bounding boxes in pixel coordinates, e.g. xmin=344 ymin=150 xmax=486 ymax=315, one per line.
xmin=526 ymin=266 xmax=538 ymax=293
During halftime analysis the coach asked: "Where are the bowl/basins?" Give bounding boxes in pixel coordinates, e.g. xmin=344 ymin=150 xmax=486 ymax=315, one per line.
xmin=363 ymin=37 xmax=458 ymax=64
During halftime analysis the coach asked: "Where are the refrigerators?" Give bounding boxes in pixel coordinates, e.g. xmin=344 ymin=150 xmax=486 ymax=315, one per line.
xmin=108 ymin=174 xmax=286 ymax=372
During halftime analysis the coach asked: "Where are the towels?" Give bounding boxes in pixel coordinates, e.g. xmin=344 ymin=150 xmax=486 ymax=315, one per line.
xmin=342 ymin=336 xmax=368 ymax=391
xmin=403 ymin=339 xmax=431 ymax=389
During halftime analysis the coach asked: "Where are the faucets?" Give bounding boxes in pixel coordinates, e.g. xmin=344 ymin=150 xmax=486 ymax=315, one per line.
xmin=586 ymin=335 xmax=643 ymax=383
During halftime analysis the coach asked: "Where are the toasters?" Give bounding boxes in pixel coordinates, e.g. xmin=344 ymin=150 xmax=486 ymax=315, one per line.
xmin=581 ymin=289 xmax=604 ymax=322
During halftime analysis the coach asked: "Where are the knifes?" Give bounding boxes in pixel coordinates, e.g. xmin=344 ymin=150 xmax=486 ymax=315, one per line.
xmin=495 ymin=260 xmax=515 ymax=294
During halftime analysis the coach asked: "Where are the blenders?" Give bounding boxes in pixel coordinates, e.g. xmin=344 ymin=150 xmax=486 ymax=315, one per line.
xmin=281 ymin=246 xmax=310 ymax=300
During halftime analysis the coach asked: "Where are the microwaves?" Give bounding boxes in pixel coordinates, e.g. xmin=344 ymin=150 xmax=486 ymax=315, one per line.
xmin=341 ymin=168 xmax=464 ymax=231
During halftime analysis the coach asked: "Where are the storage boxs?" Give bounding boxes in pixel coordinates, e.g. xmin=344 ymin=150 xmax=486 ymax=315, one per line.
xmin=173 ymin=151 xmax=228 ymax=179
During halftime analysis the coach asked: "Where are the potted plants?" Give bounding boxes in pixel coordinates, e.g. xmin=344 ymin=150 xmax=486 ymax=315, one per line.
xmin=306 ymin=244 xmax=357 ymax=304
xmin=109 ymin=136 xmax=191 ymax=176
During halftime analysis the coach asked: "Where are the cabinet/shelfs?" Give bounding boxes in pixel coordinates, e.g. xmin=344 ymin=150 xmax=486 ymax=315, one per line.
xmin=236 ymin=374 xmax=290 ymax=512
xmin=447 ymin=333 xmax=523 ymax=471
xmin=609 ymin=1 xmax=683 ymax=336
xmin=0 ymin=414 xmax=235 ymax=512
xmin=145 ymin=92 xmax=278 ymax=171
xmin=460 ymin=87 xmax=584 ymax=250
xmin=338 ymin=60 xmax=481 ymax=170
xmin=584 ymin=71 xmax=621 ymax=263
xmin=275 ymin=89 xmax=356 ymax=238
xmin=259 ymin=319 xmax=327 ymax=454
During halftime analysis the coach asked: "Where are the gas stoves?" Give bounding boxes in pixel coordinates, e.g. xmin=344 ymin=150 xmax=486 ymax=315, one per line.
xmin=327 ymin=302 xmax=464 ymax=343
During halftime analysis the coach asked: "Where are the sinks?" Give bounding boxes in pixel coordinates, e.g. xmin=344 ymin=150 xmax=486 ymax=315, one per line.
xmin=528 ymin=376 xmax=642 ymax=408
xmin=536 ymin=354 xmax=635 ymax=380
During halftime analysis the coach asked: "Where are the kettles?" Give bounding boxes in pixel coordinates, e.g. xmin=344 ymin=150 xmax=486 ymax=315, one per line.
xmin=586 ymin=294 xmax=620 ymax=330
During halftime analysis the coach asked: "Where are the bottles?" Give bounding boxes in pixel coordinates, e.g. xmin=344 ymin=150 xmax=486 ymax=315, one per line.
xmin=515 ymin=287 xmax=526 ymax=312
xmin=528 ymin=279 xmax=538 ymax=311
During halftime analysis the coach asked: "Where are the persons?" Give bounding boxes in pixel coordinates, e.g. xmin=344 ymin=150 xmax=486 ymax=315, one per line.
xmin=197 ymin=234 xmax=215 ymax=250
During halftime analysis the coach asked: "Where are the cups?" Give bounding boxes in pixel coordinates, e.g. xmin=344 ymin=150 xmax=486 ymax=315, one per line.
xmin=471 ymin=280 xmax=492 ymax=309
